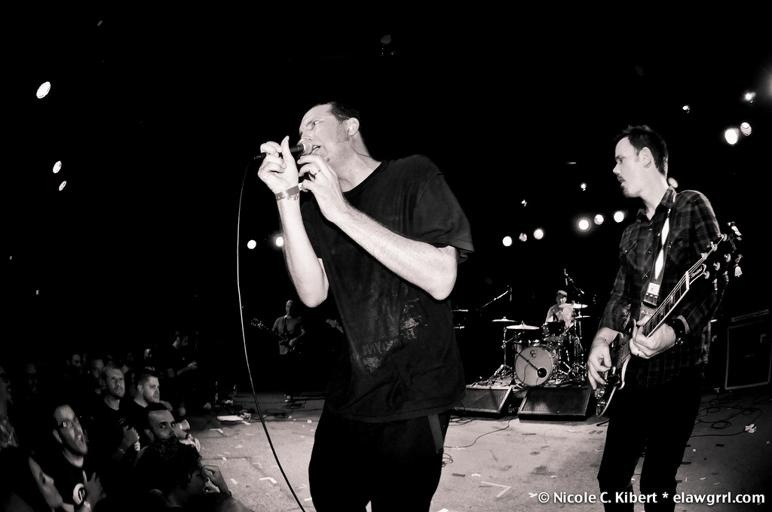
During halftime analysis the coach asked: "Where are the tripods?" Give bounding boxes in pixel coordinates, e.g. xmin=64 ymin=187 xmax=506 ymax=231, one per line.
xmin=486 ymin=327 xmax=512 ymax=385
xmin=504 ymin=343 xmax=526 ymax=388
xmin=558 ymin=312 xmax=591 ymax=387
xmin=470 ymin=312 xmax=502 ymax=387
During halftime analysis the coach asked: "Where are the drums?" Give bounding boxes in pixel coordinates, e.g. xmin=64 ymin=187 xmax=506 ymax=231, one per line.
xmin=514 ymin=343 xmax=558 ymax=386
xmin=541 ymin=319 xmax=565 ymax=337
xmin=543 ymin=334 xmax=564 ymax=365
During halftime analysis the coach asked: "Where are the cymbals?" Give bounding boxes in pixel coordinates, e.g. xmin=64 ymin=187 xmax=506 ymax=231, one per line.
xmin=559 ymin=304 xmax=587 ymax=309
xmin=572 ymin=316 xmax=590 ymax=320
xmin=492 ymin=319 xmax=516 ymax=323
xmin=506 ymin=324 xmax=539 ymax=330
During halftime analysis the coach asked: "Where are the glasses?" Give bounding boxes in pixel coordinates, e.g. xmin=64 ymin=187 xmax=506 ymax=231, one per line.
xmin=56 ymin=415 xmax=81 ymax=431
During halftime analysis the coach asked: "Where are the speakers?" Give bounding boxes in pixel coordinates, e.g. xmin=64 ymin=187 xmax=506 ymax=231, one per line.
xmin=452 ymin=386 xmax=511 ymax=415
xmin=516 ymin=388 xmax=592 ymax=419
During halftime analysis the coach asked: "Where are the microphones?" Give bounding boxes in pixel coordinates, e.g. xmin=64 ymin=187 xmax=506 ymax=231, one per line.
xmin=509 ymin=285 xmax=512 ymax=301
xmin=564 ymin=269 xmax=568 ymax=286
xmin=255 ymin=139 xmax=311 ymax=155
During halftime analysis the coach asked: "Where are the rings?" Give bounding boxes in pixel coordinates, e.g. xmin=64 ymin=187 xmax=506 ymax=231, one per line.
xmin=311 ymin=166 xmax=324 ymax=177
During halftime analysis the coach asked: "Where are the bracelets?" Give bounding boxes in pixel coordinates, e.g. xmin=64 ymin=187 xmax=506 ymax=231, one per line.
xmin=270 ymin=182 xmax=304 ymax=202
xmin=665 ymin=317 xmax=686 ymax=346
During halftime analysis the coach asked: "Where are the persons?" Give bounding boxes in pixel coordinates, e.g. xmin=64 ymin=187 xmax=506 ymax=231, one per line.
xmin=579 ymin=123 xmax=722 ymax=511
xmin=253 ymin=100 xmax=475 ymax=512
xmin=540 ymin=289 xmax=586 ymax=364
xmin=1 ymin=290 xmax=309 ymax=511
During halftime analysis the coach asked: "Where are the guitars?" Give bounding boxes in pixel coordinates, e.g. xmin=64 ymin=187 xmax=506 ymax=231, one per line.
xmin=251 ymin=318 xmax=302 ymax=350
xmin=594 ymin=222 xmax=743 ymax=418
